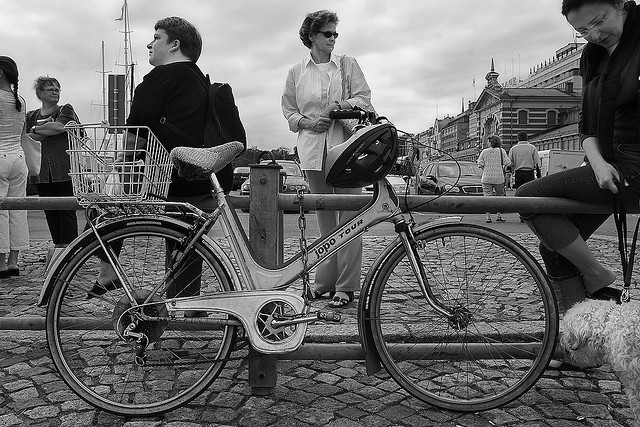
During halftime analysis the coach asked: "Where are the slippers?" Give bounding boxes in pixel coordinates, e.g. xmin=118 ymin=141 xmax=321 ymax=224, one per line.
xmin=87 ymin=280 xmax=124 ymax=299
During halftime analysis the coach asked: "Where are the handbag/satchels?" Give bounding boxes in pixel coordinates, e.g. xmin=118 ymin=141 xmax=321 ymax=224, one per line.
xmin=19 ymin=95 xmax=42 ymax=177
xmin=338 ymin=56 xmax=380 ymax=136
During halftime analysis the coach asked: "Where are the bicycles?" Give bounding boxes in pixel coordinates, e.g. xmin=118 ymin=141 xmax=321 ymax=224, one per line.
xmin=37 ymin=105 xmax=558 ymax=416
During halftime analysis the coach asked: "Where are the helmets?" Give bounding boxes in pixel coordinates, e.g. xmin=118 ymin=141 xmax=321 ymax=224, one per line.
xmin=325 ymin=123 xmax=400 ymax=189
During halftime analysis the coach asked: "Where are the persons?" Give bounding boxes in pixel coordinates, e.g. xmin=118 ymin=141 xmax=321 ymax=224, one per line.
xmin=85 ymin=17 xmax=248 ymax=319
xmin=478 ymin=135 xmax=513 ymax=224
xmin=0 ymin=56 xmax=31 ymax=278
xmin=22 ymin=77 xmax=85 ymax=246
xmin=516 ymin=1 xmax=639 ymax=368
xmin=281 ymin=10 xmax=382 ymax=308
xmin=508 ymin=131 xmax=543 ymax=222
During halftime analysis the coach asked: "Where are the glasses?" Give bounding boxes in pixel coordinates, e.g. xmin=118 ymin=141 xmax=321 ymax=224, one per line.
xmin=574 ymin=4 xmax=614 ymax=39
xmin=43 ymin=89 xmax=61 ymax=94
xmin=318 ymin=31 xmax=339 ymax=39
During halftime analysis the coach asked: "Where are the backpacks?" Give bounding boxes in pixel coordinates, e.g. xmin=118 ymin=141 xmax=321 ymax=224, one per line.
xmin=160 ymin=62 xmax=247 ymax=159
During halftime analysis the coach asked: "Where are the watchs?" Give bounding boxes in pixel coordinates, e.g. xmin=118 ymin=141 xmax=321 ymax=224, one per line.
xmin=31 ymin=125 xmax=37 ymax=134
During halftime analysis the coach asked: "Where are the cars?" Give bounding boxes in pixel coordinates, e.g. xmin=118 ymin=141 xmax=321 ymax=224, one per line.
xmin=240 ymin=159 xmax=310 ymax=211
xmin=365 ymin=176 xmax=409 ymax=194
xmin=232 ymin=165 xmax=249 ymax=189
xmin=417 ymin=160 xmax=505 ymax=195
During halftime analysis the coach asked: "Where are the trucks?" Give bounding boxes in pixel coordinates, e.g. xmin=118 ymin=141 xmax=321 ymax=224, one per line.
xmin=509 ymin=148 xmax=584 ymax=190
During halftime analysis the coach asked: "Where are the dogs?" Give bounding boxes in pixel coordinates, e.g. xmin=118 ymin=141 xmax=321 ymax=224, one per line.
xmin=558 ymin=298 xmax=640 ymax=425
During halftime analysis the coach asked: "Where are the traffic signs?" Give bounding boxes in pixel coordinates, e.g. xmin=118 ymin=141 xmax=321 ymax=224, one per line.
xmin=108 ymin=74 xmax=125 ymax=133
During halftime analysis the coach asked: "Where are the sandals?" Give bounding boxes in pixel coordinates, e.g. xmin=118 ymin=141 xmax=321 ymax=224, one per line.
xmin=302 ymin=288 xmax=336 ymax=300
xmin=330 ymin=292 xmax=355 ymax=308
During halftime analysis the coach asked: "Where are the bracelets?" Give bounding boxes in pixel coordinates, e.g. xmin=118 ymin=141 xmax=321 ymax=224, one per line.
xmin=335 ymin=101 xmax=342 ymax=110
